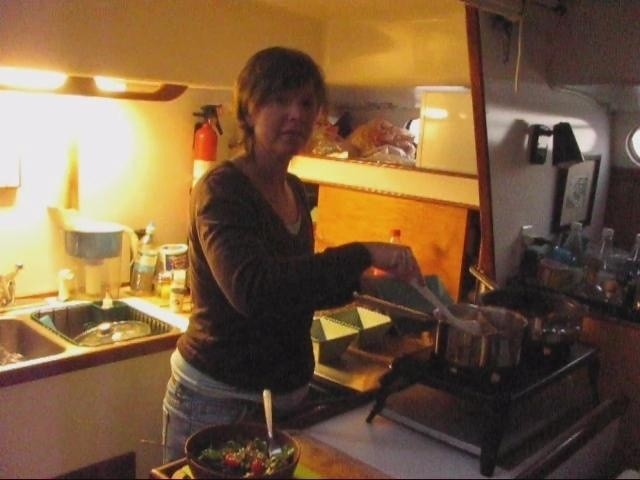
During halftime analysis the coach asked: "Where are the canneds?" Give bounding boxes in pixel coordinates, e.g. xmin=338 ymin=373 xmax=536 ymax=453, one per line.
xmin=169 ymin=288 xmax=185 ymax=311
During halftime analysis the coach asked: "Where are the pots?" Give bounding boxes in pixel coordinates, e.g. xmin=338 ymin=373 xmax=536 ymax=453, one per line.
xmin=59 ymin=221 xmax=128 ymax=261
xmin=432 ymin=302 xmax=528 ymax=375
xmin=467 ymin=264 xmax=590 ymax=350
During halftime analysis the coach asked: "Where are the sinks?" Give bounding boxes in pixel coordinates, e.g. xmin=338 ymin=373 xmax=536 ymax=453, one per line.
xmin=30 ymin=296 xmax=190 ymax=348
xmin=0 ymin=308 xmax=69 ymax=370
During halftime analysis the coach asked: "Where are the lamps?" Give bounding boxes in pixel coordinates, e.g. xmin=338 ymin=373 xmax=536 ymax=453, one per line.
xmin=526 ymin=122 xmax=586 ymax=170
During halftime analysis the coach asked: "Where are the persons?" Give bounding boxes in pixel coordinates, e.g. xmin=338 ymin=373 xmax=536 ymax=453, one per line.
xmin=162 ymin=47 xmax=425 ymax=463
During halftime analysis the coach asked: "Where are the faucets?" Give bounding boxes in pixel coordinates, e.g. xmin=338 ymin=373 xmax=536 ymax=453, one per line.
xmin=58 ymin=269 xmax=75 ymax=301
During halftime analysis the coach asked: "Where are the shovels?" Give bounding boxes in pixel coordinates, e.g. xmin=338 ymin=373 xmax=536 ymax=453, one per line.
xmin=409 ymin=276 xmax=482 ymax=334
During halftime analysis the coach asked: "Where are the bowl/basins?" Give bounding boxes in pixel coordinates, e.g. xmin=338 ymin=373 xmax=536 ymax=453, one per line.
xmin=179 ymin=424 xmax=299 ymax=479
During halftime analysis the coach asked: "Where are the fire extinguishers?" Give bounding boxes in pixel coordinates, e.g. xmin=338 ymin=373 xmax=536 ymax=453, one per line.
xmin=191 ymin=104 xmax=223 ymax=194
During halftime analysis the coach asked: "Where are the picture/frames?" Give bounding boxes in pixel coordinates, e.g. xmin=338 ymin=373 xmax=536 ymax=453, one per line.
xmin=553 ymin=154 xmax=602 ymax=232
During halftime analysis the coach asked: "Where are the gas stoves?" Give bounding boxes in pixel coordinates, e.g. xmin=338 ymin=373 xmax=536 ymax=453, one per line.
xmin=360 ymin=345 xmax=603 ymax=476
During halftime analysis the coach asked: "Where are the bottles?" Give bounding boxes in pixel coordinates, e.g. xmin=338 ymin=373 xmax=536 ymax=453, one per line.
xmin=387 ymin=229 xmax=403 ymax=245
xmin=624 ymin=233 xmax=639 ymax=263
xmin=566 ymin=221 xmax=587 ymax=255
xmin=132 ymin=223 xmax=158 ymax=292
xmin=591 ymin=228 xmax=613 ymax=263
